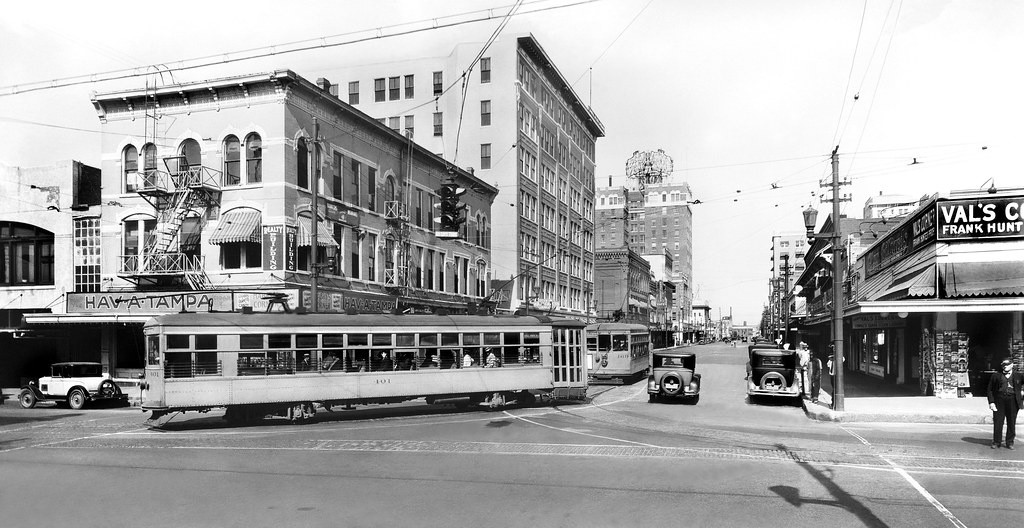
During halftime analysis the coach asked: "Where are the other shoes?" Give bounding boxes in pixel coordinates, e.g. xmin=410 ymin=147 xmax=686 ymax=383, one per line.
xmin=1007 ymin=445 xmax=1015 ymax=450
xmin=991 ymin=444 xmax=999 ymax=449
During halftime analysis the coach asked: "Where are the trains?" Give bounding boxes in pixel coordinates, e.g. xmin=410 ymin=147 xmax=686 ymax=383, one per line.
xmin=585 ymin=282 xmax=650 ymax=385
xmin=141 ymin=247 xmax=588 ymax=430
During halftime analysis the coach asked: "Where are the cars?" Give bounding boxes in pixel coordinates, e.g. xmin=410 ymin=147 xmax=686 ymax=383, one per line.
xmin=720 ymin=336 xmax=802 ymax=404
xmin=648 ymin=351 xmax=700 ymax=404
xmin=18 ymin=362 xmax=115 ymax=409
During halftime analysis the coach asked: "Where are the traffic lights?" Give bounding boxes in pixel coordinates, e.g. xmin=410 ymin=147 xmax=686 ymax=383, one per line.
xmin=434 ymin=183 xmax=467 ymax=240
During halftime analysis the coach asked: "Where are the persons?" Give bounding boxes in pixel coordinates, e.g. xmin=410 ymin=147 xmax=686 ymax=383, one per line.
xmin=826 ymin=354 xmax=846 ymax=406
xmin=145 ymin=337 xmax=541 ymax=378
xmin=716 ymin=331 xmax=823 ymax=403
xmin=604 ymin=339 xmax=675 ymax=366
xmin=987 ymin=357 xmax=1024 ymax=450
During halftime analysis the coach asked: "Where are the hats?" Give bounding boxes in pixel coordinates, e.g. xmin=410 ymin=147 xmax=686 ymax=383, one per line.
xmin=1000 ymin=358 xmax=1013 ymax=367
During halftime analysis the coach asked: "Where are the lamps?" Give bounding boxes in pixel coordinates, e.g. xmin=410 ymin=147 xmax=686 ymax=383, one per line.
xmin=980 ymin=177 xmax=997 ymax=195
